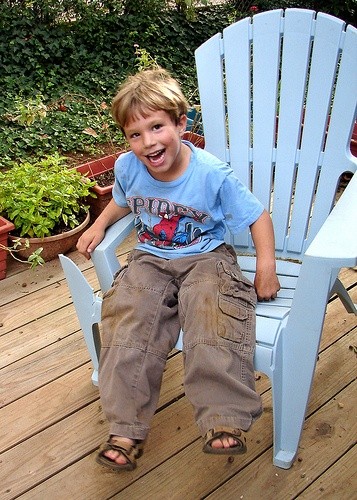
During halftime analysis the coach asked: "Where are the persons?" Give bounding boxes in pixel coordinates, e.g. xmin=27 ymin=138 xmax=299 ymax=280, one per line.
xmin=76 ymin=69 xmax=281 ymax=471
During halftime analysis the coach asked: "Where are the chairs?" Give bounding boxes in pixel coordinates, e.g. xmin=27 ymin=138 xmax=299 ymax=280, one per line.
xmin=58 ymin=8 xmax=357 ymax=470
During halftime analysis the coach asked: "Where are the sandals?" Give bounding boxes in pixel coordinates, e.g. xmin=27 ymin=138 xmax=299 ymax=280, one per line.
xmin=202 ymin=416 xmax=247 ymax=455
xmin=95 ymin=434 xmax=144 ymax=471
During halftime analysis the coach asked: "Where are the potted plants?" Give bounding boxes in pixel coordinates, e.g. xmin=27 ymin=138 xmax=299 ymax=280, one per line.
xmin=70 ymin=102 xmax=207 ymax=218
xmin=0 ymin=155 xmax=97 ymax=263
xmin=0 ymin=216 xmax=44 ymax=280
